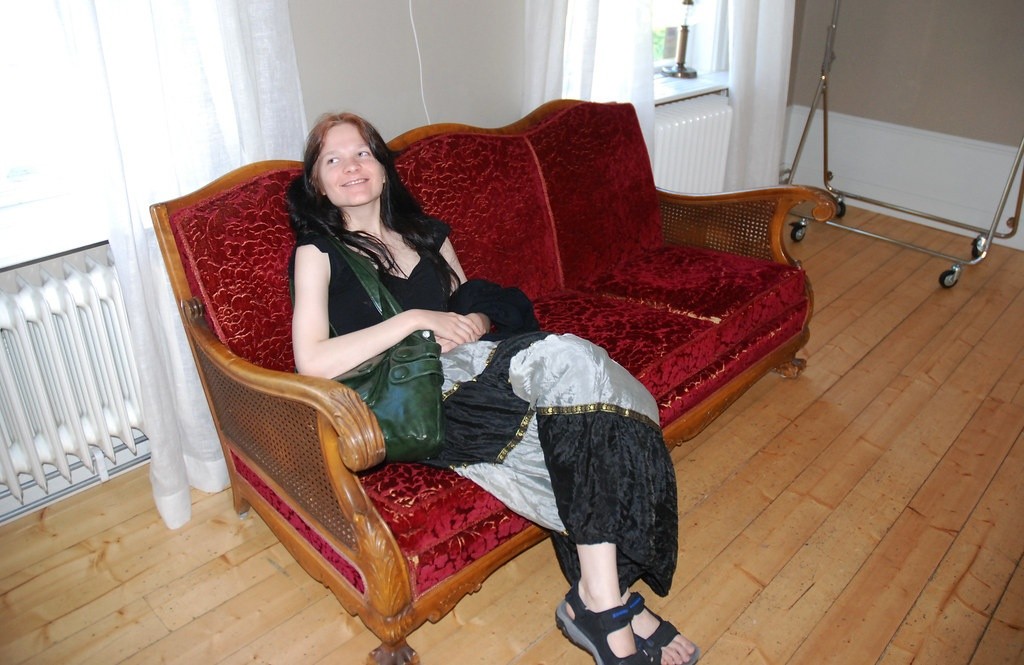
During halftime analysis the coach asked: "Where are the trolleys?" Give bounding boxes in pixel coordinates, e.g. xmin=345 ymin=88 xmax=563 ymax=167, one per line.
xmin=777 ymin=0 xmax=1024 ymax=291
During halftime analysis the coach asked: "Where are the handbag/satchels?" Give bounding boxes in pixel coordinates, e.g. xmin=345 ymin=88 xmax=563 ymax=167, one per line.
xmin=289 ymin=237 xmax=445 ymax=463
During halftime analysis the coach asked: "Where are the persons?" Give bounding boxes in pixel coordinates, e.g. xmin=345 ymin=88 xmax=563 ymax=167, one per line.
xmin=289 ymin=111 xmax=700 ymax=665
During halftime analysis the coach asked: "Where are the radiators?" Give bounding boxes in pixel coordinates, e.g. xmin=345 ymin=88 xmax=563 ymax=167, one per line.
xmin=0 ymin=248 xmax=151 ymax=504
xmin=654 ymin=95 xmax=734 ymax=194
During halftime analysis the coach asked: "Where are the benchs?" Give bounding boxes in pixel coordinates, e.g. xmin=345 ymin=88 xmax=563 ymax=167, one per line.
xmin=149 ymin=100 xmax=839 ymax=665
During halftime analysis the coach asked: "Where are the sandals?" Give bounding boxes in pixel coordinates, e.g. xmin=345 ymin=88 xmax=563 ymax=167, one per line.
xmin=555 ymin=581 xmax=649 ymax=665
xmin=624 ymin=591 xmax=700 ymax=665
